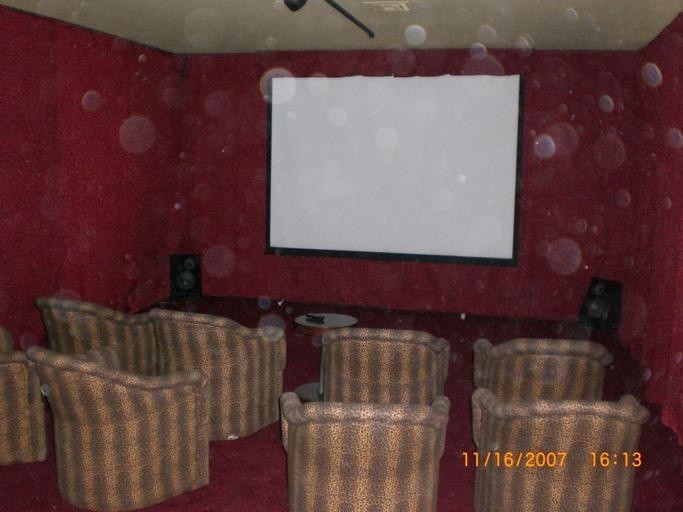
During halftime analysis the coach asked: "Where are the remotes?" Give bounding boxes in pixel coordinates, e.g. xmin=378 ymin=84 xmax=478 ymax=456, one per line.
xmin=305 ymin=312 xmax=326 ymax=321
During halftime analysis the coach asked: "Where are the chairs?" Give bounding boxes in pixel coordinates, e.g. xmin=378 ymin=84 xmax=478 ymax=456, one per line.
xmin=36 ymin=292 xmax=149 ymax=375
xmin=471 ymin=388 xmax=647 ymax=512
xmin=146 ymin=309 xmax=287 ymax=439
xmin=1 ymin=324 xmax=48 ymax=463
xmin=476 ymin=337 xmax=616 ymax=408
xmin=27 ymin=344 xmax=211 ymax=512
xmin=322 ymin=326 xmax=449 ymax=405
xmin=280 ymin=392 xmax=450 ymax=510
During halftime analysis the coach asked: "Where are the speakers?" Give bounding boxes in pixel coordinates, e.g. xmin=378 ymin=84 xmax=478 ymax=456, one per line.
xmin=577 ymin=277 xmax=623 ymax=332
xmin=170 ymin=253 xmax=203 ymax=301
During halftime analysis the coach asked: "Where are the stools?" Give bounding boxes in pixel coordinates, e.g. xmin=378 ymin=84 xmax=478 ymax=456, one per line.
xmin=295 ymin=310 xmax=358 ymax=404
